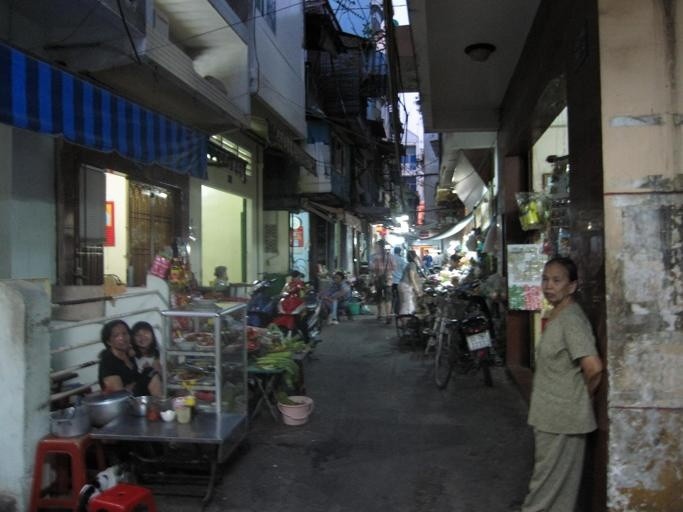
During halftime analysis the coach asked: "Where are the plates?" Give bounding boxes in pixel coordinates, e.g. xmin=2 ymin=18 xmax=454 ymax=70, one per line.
xmin=169 ymin=361 xmax=239 ymax=385
xmin=192 ymin=297 xmax=220 ymax=305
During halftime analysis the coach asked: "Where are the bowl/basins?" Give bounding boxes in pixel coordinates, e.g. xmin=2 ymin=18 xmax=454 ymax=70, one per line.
xmin=133 ymin=394 xmax=218 ymax=426
xmin=174 ymin=328 xmax=238 ymax=350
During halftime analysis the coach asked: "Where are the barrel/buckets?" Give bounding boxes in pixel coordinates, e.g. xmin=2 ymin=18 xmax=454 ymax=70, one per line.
xmin=276 ymin=395 xmax=315 ymax=427
xmin=347 ymin=302 xmax=361 ymax=315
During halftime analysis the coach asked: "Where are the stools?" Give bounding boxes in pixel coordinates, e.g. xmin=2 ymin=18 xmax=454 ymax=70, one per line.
xmin=87 ymin=483 xmax=156 ymax=511
xmin=29 ymin=434 xmax=104 ymax=511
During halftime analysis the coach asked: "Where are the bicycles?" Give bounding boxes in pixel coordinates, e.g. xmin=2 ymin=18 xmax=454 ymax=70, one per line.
xmin=423 ymin=282 xmax=462 ymax=392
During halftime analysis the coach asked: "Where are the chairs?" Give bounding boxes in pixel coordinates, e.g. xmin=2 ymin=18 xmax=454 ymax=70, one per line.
xmin=336 ymin=287 xmax=353 ymax=322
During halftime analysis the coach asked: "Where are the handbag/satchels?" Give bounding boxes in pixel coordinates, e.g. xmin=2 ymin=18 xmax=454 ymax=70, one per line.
xmin=375 ymin=275 xmax=386 ymax=291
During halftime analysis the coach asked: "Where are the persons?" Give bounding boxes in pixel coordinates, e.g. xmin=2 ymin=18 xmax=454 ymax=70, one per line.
xmin=127 ymin=320 xmax=163 ymax=381
xmin=323 ymin=237 xmax=444 ymax=336
xmin=521 ymin=255 xmax=604 ymax=512
xmin=97 ymin=319 xmax=161 ymax=397
xmin=213 ymin=265 xmax=229 ymax=296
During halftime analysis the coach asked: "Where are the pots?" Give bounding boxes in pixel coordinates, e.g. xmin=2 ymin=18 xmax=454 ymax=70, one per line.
xmin=48 ymin=389 xmax=133 ymax=438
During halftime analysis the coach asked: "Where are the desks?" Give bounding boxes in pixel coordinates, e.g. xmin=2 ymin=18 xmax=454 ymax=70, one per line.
xmin=242 ymin=343 xmax=310 ymax=426
xmin=89 ymin=408 xmax=247 ymax=509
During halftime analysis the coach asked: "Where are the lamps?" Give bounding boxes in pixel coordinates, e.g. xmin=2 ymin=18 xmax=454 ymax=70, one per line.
xmin=464 ymin=42 xmax=497 ymax=63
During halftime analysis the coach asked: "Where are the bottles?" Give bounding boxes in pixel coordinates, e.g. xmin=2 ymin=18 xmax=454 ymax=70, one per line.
xmin=168 ymin=257 xmax=185 ymax=282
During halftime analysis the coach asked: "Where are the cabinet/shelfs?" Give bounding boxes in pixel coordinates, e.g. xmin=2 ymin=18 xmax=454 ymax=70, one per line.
xmin=157 ymin=301 xmax=250 ymax=467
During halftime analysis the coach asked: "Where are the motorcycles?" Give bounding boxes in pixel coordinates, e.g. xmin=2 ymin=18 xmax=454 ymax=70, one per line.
xmin=244 ymin=277 xmax=278 ymax=328
xmin=448 ymin=279 xmax=499 ymax=387
xmin=273 ymin=278 xmax=321 ymax=345
